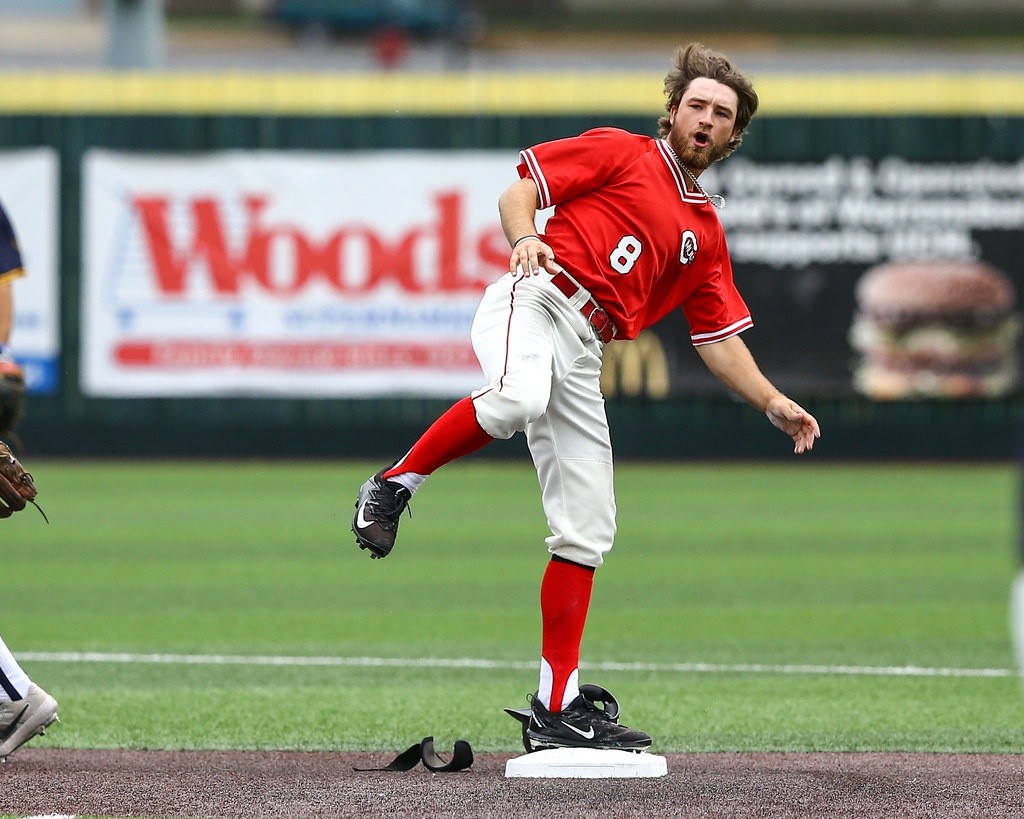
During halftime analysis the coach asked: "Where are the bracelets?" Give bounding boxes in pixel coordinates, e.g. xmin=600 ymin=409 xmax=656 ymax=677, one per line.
xmin=514 ymin=234 xmax=542 ymax=247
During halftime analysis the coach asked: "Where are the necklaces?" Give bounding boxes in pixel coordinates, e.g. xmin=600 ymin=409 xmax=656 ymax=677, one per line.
xmin=664 ymin=140 xmax=726 ymax=209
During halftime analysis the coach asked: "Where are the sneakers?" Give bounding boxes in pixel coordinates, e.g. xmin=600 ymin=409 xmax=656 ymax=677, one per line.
xmin=350 ymin=459 xmax=412 ymax=559
xmin=504 ymin=688 xmax=652 ymax=754
xmin=0 ymin=685 xmax=60 ymax=761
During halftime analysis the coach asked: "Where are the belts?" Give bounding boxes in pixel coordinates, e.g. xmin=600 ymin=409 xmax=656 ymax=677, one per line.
xmin=548 ymin=271 xmax=618 ymax=343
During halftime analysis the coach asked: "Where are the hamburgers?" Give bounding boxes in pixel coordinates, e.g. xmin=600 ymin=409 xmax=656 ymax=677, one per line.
xmin=847 ymin=257 xmax=1016 ymax=400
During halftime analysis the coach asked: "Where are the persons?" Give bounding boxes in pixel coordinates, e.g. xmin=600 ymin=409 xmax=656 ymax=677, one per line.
xmin=350 ymin=41 xmax=822 ymax=755
xmin=0 ymin=198 xmax=60 ymax=763
